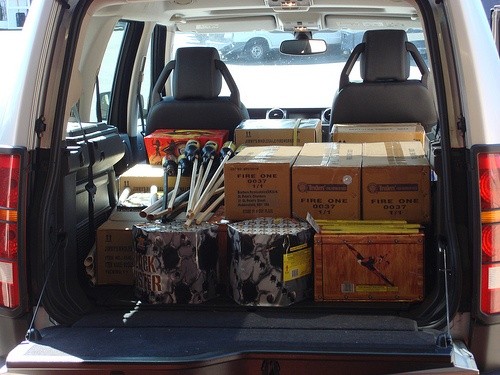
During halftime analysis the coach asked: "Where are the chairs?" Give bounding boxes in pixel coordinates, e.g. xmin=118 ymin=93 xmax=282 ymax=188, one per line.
xmin=145 ymin=47 xmax=250 ymax=142
xmin=329 ymin=29 xmax=438 ymax=133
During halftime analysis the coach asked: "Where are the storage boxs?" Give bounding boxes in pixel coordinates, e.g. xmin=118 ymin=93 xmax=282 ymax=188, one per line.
xmin=292 ymin=142 xmax=362 ymax=220
xmin=96 ymin=212 xmax=214 ymax=286
xmin=223 ymin=145 xmax=303 ymax=221
xmin=119 ymin=164 xmax=191 ymax=193
xmin=144 ymin=129 xmax=229 ymax=166
xmin=313 ymin=233 xmax=425 ymax=303
xmin=361 ymin=140 xmax=431 ymax=225
xmin=331 ymin=122 xmax=426 ymax=144
xmin=234 ymin=118 xmax=322 ymax=146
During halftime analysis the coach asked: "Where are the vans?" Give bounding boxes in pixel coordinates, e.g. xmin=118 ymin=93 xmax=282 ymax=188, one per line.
xmin=0 ymin=0 xmax=500 ymax=375
